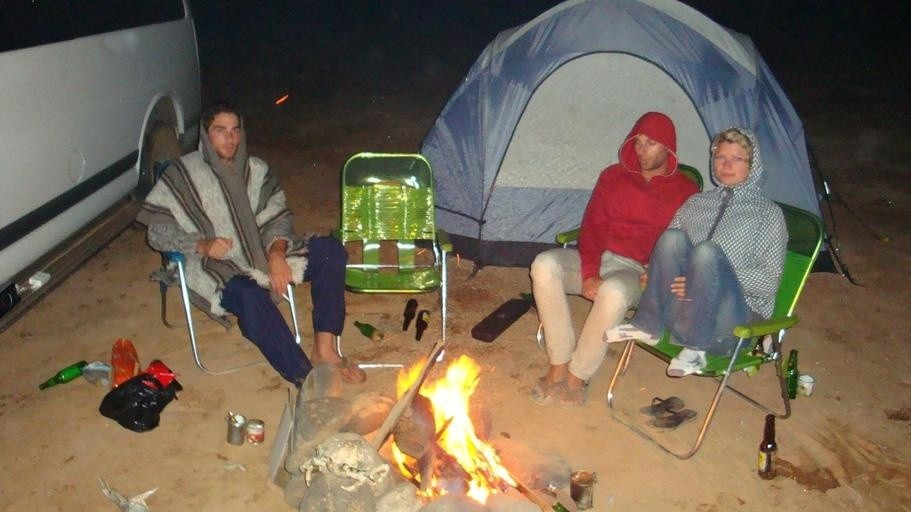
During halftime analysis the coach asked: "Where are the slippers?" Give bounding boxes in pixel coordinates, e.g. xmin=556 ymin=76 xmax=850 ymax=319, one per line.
xmin=645 ymin=408 xmax=699 ymax=431
xmin=531 ymin=368 xmax=569 ymax=407
xmin=558 ymin=369 xmax=591 ymax=408
xmin=311 ymin=354 xmax=368 ymax=385
xmin=639 ymin=394 xmax=686 ymax=417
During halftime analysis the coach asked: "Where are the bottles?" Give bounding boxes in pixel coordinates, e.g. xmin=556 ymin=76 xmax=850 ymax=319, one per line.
xmin=38 ymin=359 xmax=88 ymax=390
xmin=246 ymin=419 xmax=265 ymax=445
xmin=780 ymin=349 xmax=799 ymax=400
xmin=756 ymin=414 xmax=780 ymax=482
xmin=352 ymin=320 xmax=384 ymax=344
xmin=402 ymin=299 xmax=430 ymax=341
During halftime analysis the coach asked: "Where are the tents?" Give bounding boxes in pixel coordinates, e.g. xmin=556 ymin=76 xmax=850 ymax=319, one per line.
xmin=409 ymin=1 xmax=840 ymax=281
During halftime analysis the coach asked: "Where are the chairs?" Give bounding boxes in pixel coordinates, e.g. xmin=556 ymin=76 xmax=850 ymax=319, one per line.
xmin=557 ymin=161 xmax=705 ymax=374
xmin=328 ymin=153 xmax=454 ymax=369
xmin=153 ymin=159 xmax=299 ymax=376
xmin=606 ymin=200 xmax=825 ymax=460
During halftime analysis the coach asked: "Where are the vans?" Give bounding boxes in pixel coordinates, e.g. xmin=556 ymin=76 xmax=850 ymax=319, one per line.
xmin=0 ymin=0 xmax=204 ymax=333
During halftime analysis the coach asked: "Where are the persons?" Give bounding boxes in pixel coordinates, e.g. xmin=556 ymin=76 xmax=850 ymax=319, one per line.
xmin=603 ymin=127 xmax=790 ymax=378
xmin=529 ymin=112 xmax=700 ymax=408
xmin=134 ymin=102 xmax=367 ymax=393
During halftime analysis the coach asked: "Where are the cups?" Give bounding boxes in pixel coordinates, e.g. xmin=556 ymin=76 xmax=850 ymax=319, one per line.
xmin=570 ymin=471 xmax=595 ymax=510
xmin=226 ymin=413 xmax=246 ymax=447
xmin=795 ymin=373 xmax=815 ymax=397
